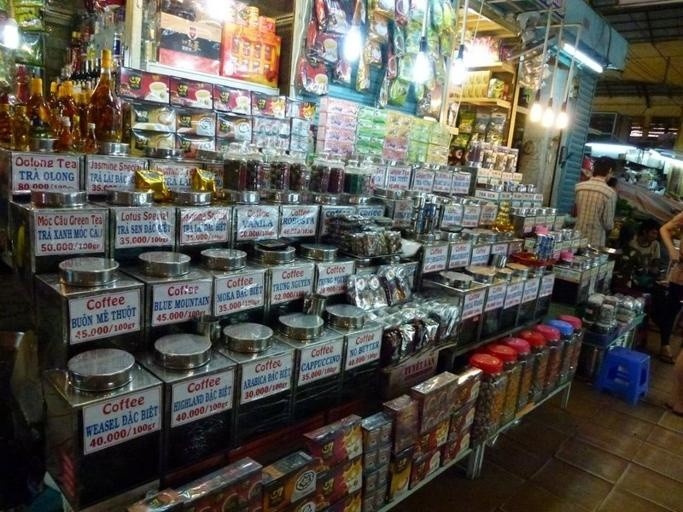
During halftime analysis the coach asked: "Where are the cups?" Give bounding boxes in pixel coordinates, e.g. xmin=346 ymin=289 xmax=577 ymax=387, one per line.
xmin=235 ymin=96 xmax=250 ymax=108
xmin=194 ymin=89 xmax=212 ymax=105
xmin=148 ymin=81 xmax=169 ymax=98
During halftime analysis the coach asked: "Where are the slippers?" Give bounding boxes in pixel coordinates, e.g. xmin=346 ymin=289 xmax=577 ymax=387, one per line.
xmin=662 ymin=402 xmax=682 ymax=417
xmin=657 ymin=352 xmax=676 ymax=365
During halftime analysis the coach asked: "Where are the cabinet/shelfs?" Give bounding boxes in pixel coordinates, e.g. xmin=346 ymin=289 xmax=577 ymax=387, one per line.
xmin=2 ymin=144 xmax=650 ymax=511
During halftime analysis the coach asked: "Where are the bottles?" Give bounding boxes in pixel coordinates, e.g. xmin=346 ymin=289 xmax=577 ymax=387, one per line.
xmin=223 ymin=140 xmax=368 ymax=195
xmin=461 ymin=313 xmax=584 ymax=450
xmin=4 ymin=44 xmax=124 ymax=152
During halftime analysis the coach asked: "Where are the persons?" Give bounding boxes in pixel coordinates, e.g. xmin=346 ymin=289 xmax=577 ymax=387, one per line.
xmin=660 ymin=351 xmax=682 ymax=418
xmin=631 ymin=217 xmax=661 ymax=260
xmin=659 ymin=212 xmax=683 ymax=365
xmin=572 ymin=154 xmax=619 ymax=248
